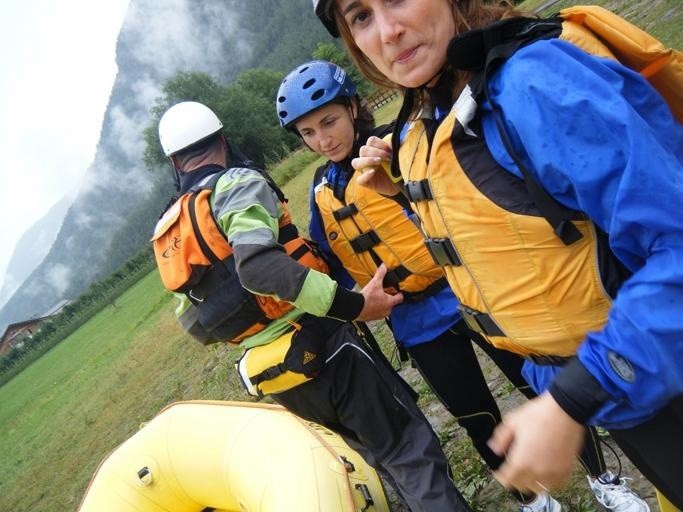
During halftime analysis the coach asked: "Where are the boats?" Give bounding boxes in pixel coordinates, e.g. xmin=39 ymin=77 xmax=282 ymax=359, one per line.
xmin=77 ymin=399 xmax=393 ymax=511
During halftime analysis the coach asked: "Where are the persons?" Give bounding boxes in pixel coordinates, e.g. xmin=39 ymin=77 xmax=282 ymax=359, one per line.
xmin=310 ymin=0 xmax=683 ymax=512
xmin=275 ymin=61 xmax=650 ymax=512
xmin=150 ymin=101 xmax=471 ymax=511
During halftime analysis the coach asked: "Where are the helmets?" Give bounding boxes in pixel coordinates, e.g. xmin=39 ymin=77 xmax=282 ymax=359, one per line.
xmin=156 ymin=101 xmax=223 ymax=157
xmin=276 ymin=60 xmax=359 ymax=135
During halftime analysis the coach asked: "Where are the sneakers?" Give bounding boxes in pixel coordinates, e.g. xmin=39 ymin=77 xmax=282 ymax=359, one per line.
xmin=585 ymin=470 xmax=652 ymax=512
xmin=522 ymin=490 xmax=564 ymax=512
